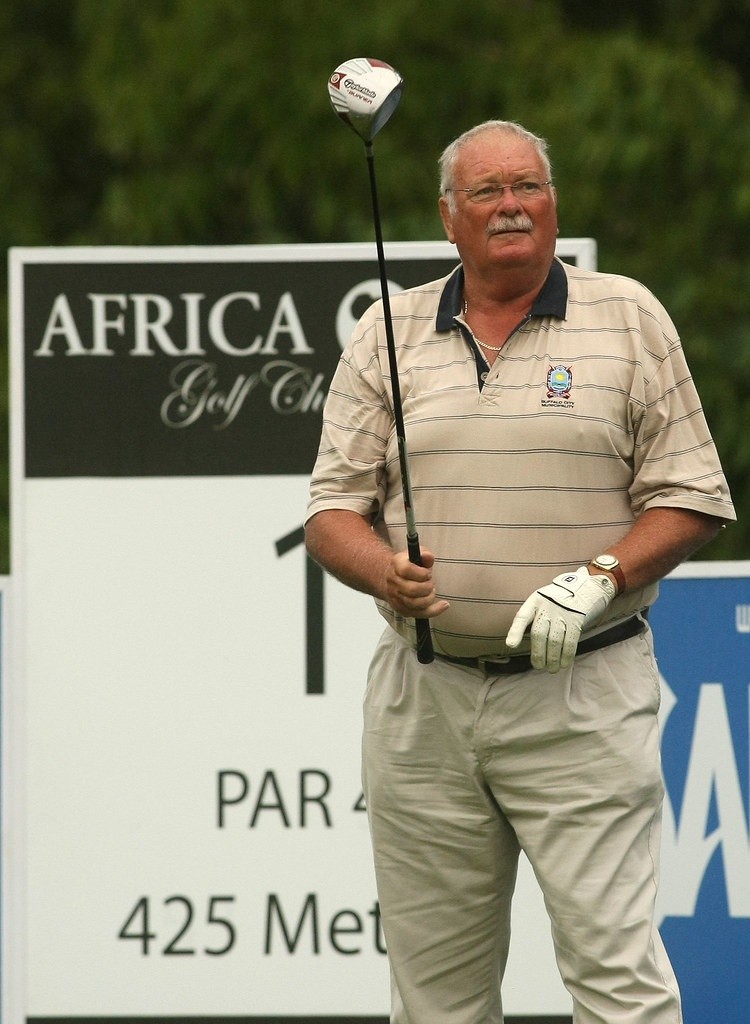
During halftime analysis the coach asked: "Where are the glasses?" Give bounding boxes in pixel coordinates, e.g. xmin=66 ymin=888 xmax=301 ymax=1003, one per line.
xmin=444 ymin=179 xmax=551 ymax=204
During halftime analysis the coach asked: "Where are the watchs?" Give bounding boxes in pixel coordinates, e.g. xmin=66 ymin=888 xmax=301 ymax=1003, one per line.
xmin=589 ymin=554 xmax=627 ymax=598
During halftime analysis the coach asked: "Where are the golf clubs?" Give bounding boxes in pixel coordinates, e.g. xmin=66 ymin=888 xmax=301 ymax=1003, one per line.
xmin=327 ymin=57 xmax=435 ymax=665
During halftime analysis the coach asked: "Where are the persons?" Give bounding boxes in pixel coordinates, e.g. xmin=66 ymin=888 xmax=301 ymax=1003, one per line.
xmin=307 ymin=117 xmax=736 ymax=1024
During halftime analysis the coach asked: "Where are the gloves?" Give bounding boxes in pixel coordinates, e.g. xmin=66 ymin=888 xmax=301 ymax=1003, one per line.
xmin=505 ymin=566 xmax=614 ymax=673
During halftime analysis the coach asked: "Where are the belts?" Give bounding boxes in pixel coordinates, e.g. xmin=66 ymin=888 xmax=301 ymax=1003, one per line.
xmin=434 ymin=607 xmax=649 ymax=676
